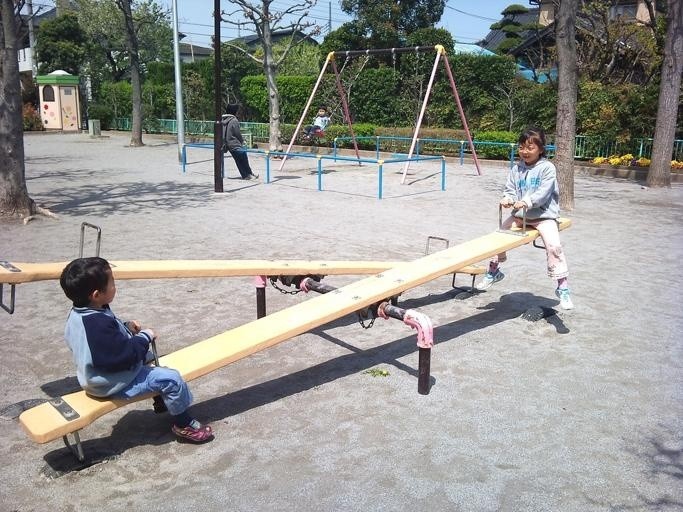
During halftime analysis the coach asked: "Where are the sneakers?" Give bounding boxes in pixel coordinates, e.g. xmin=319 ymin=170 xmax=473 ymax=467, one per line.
xmin=244 ymin=173 xmax=258 ymax=180
xmin=555 ymin=287 xmax=573 ymax=310
xmin=153 ymin=399 xmax=168 ymax=413
xmin=477 ymin=267 xmax=505 ymax=291
xmin=172 ymin=418 xmax=212 ymax=441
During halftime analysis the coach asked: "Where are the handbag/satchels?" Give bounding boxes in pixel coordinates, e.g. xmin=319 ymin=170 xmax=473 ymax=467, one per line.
xmin=221 ymin=144 xmax=228 ymax=153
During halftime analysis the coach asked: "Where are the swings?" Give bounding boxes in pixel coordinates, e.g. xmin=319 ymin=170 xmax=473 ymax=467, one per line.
xmin=301 ymin=55 xmax=370 ymax=137
xmin=393 ymin=53 xmax=419 ymax=159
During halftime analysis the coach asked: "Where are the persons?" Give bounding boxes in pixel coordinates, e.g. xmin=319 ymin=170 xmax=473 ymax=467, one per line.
xmin=221 ymin=103 xmax=259 ymax=181
xmin=476 ymin=126 xmax=574 ymax=311
xmin=59 ymin=256 xmax=213 ymax=442
xmin=300 ymin=104 xmax=333 ymax=145
